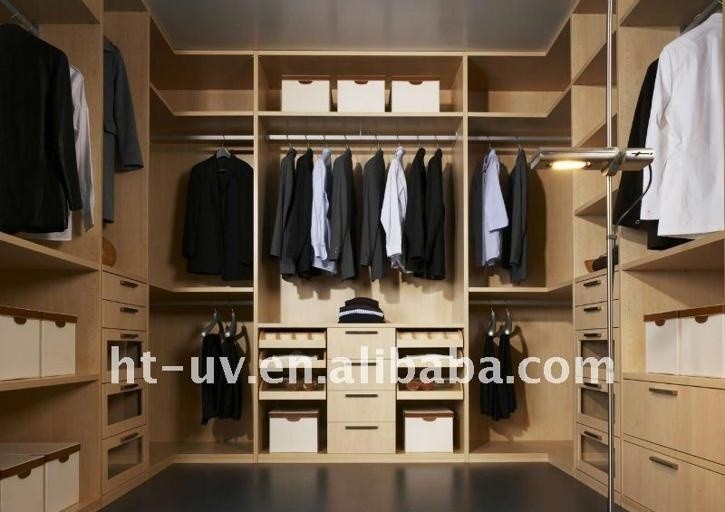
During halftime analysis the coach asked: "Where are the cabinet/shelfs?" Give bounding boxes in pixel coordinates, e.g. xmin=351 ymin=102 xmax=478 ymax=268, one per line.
xmin=0 ymin=0 xmax=724 ymax=512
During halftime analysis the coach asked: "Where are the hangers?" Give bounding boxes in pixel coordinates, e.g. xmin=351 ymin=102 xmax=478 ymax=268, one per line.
xmin=212 ymin=132 xmax=526 ymax=160
